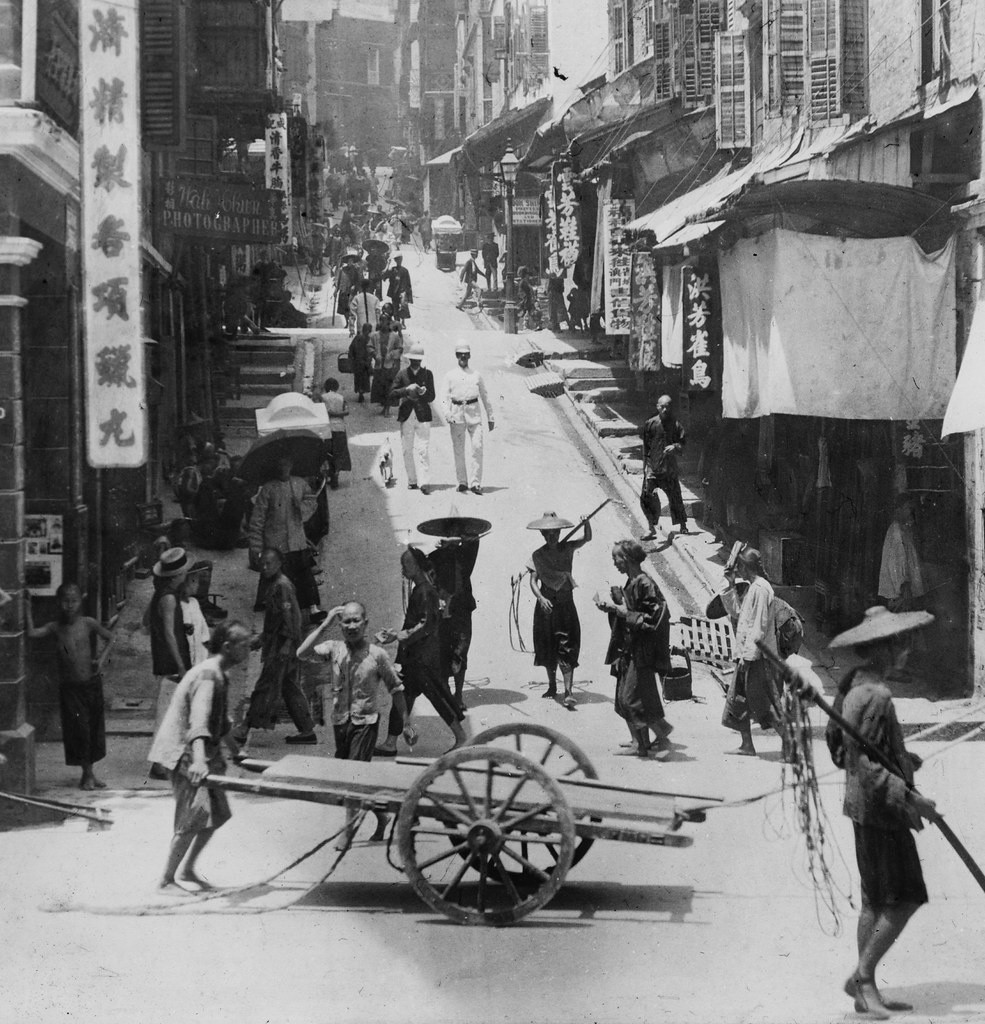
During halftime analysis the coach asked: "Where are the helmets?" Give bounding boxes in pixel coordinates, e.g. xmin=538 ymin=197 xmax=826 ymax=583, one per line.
xmin=455 ymin=344 xmax=471 ymax=358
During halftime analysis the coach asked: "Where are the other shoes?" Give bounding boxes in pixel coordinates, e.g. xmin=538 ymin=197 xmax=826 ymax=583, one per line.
xmin=407 ymin=483 xmax=418 ymax=490
xmin=233 ymin=736 xmax=246 ymax=747
xmin=471 ymin=485 xmax=482 ymax=495
xmin=285 ymin=733 xmax=317 ymax=745
xmin=456 ymin=484 xmax=468 ymax=492
xmin=421 ymin=484 xmax=429 ymax=495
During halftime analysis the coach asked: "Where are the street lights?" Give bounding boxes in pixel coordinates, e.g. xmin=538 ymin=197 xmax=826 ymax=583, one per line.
xmin=500 ymin=137 xmax=521 ymax=334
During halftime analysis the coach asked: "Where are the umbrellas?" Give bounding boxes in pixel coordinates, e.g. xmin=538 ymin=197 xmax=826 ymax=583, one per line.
xmin=232 ymin=429 xmax=328 ymax=481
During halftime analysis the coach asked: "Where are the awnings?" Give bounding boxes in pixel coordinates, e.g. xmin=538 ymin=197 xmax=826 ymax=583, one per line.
xmin=424 ymin=141 xmax=521 ymax=168
xmin=452 ymin=88 xmax=559 ymax=178
xmin=620 ymin=82 xmax=976 ymax=250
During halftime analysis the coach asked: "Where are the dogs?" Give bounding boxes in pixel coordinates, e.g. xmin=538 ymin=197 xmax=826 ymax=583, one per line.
xmin=377 ymin=435 xmax=393 ymax=487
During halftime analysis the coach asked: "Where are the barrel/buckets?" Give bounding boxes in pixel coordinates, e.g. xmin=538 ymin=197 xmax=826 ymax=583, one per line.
xmin=659 ymin=646 xmax=692 ymax=700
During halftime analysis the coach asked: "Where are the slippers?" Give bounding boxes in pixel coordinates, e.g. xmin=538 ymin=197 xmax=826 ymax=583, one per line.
xmin=374 ymin=748 xmax=397 ymax=757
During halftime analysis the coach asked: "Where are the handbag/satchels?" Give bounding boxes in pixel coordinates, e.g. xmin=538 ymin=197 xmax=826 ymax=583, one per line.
xmin=337 ymin=353 xmax=351 ymax=374
xmin=824 ymin=667 xmax=867 ymax=770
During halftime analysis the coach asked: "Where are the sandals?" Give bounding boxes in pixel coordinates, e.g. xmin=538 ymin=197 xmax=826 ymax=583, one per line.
xmin=853 ymin=998 xmax=914 ymax=1011
xmin=846 ymin=969 xmax=891 ymax=1019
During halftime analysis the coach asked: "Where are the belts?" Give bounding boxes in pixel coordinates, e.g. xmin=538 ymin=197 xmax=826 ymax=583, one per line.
xmin=451 ymin=397 xmax=479 ymax=405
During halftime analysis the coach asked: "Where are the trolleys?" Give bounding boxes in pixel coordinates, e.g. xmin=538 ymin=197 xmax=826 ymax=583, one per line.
xmin=188 ymin=722 xmax=705 ymax=926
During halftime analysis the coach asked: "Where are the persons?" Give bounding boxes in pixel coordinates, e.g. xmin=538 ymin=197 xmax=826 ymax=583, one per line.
xmin=524 ymin=510 xmax=592 ymax=708
xmin=876 ymin=492 xmax=927 ymax=685
xmin=826 ymin=602 xmax=937 ymax=1023
xmin=247 ymin=247 xmax=273 ymax=332
xmin=147 ymin=622 xmax=255 ymax=902
xmin=385 ymin=545 xmax=466 ymax=755
xmin=698 ymin=409 xmax=759 ymax=540
xmin=25 ymin=583 xmax=118 ymax=793
xmin=230 ymin=547 xmax=318 ymax=747
xmin=141 ymin=546 xmax=211 ymax=782
xmin=248 ymin=443 xmax=329 ymax=625
xmin=594 ymin=539 xmax=674 ymax=761
xmin=297 ymin=598 xmax=408 ymax=851
xmin=390 ymin=343 xmax=435 ymax=495
xmin=704 ymin=546 xmax=806 ymax=763
xmin=175 ymin=410 xmax=249 ymax=553
xmin=637 ymin=394 xmax=690 ymax=542
xmin=416 ymin=506 xmax=492 ymax=717
xmin=443 ymin=339 xmax=494 ymax=497
xmin=317 ymin=373 xmax=348 ymax=491
xmin=301 ymin=123 xmax=590 ymax=417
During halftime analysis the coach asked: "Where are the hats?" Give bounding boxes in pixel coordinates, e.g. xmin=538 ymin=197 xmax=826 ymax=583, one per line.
xmin=526 ymin=512 xmax=574 ymax=529
xmin=153 ymin=548 xmax=196 ymax=577
xmin=829 ymin=607 xmax=935 ymax=648
xmin=705 ymin=578 xmax=750 ymax=620
xmin=402 ymin=343 xmax=427 ymax=360
xmin=341 ymin=252 xmax=359 ymax=264
xmin=417 ymin=507 xmax=492 ymax=538
